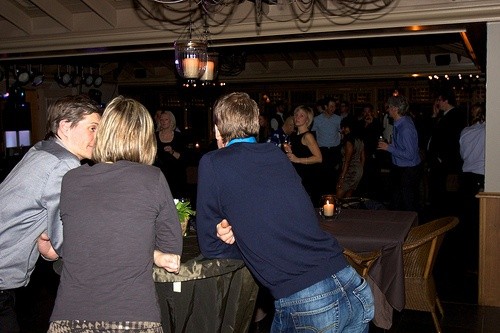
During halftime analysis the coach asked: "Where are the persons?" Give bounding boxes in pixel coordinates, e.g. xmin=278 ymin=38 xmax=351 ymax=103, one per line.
xmin=196 ymin=91 xmax=376 ymax=333
xmin=260 ymin=86 xmax=487 ymax=183
xmin=152 ymin=110 xmax=181 ymax=134
xmin=267 ymin=115 xmax=298 ymax=149
xmin=376 ymin=95 xmax=422 ymax=214
xmin=257 ymin=113 xmax=270 ymax=140
xmin=429 ymin=89 xmax=468 ymax=219
xmin=45 ymin=96 xmax=184 ymax=333
xmin=308 ymin=98 xmax=344 ymax=192
xmin=153 ymin=109 xmax=189 ymax=205
xmin=280 ymin=104 xmax=325 ymax=207
xmin=445 ymin=118 xmax=486 ymax=274
xmin=0 ymin=95 xmax=104 ymax=333
xmin=336 ymin=116 xmax=380 ymax=208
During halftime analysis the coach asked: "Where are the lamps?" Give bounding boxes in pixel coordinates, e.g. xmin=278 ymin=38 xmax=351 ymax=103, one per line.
xmin=7 ymin=62 xmax=104 ymax=87
xmin=196 ymin=0 xmax=219 ymax=87
xmin=173 ymin=0 xmax=208 ymax=87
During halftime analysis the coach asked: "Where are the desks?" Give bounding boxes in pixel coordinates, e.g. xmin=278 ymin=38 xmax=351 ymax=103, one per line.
xmin=53 ymin=222 xmax=246 ymax=333
xmin=311 ymin=207 xmax=419 ymax=333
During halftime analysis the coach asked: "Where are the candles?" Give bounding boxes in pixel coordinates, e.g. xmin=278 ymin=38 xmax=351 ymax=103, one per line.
xmin=323 ymin=200 xmax=334 ymax=216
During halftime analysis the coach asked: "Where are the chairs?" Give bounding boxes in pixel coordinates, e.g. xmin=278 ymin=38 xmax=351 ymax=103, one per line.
xmin=401 ymin=216 xmax=459 ymax=333
xmin=335 ymin=197 xmax=387 ymax=211
xmin=341 ymin=247 xmax=383 ymax=280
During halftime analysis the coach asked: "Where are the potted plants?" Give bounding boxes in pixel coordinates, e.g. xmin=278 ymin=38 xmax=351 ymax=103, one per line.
xmin=174 ymin=199 xmax=197 ymax=237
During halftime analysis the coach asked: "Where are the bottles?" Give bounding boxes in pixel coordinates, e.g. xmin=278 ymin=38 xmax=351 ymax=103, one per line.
xmin=377 ymin=136 xmax=384 ymax=142
